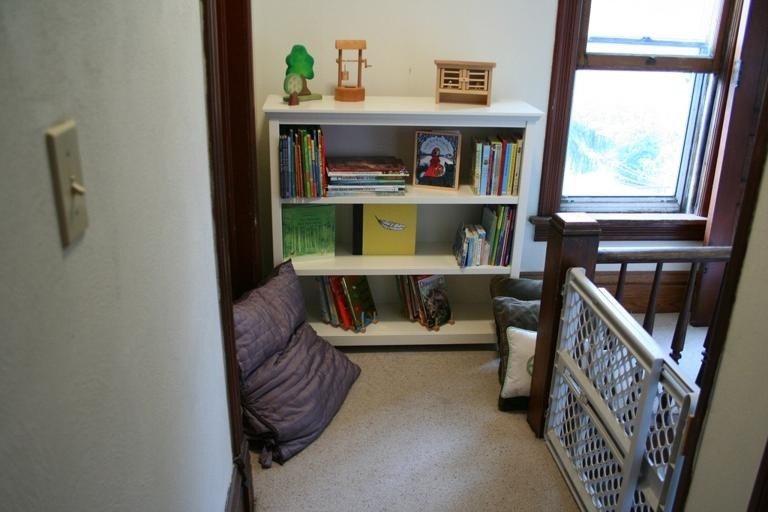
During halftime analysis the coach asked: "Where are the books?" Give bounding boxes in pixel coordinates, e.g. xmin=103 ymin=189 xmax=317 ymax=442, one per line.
xmin=316 ymin=274 xmax=377 ymax=333
xmin=353 ymin=204 xmax=417 ymax=257
xmin=278 ymin=127 xmax=410 ymax=200
xmin=396 ymin=274 xmax=453 ymax=329
xmin=452 ymin=203 xmax=517 ymax=269
xmin=282 ymin=204 xmax=337 ymax=262
xmin=469 ymin=132 xmax=524 ymax=196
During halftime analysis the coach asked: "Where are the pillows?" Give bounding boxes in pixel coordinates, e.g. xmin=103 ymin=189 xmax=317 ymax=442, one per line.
xmin=501 ymin=327 xmax=537 ymax=398
xmin=492 ymin=296 xmax=544 ymax=411
xmin=231 ymin=258 xmax=361 ymax=468
xmin=490 ymin=273 xmax=542 ymax=355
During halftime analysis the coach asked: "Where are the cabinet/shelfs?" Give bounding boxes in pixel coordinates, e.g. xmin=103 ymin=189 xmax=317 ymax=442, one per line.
xmin=262 ymin=93 xmax=545 ymax=345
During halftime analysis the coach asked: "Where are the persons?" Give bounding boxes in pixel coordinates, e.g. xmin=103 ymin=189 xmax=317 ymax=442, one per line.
xmin=425 ymin=146 xmax=444 ymax=176
